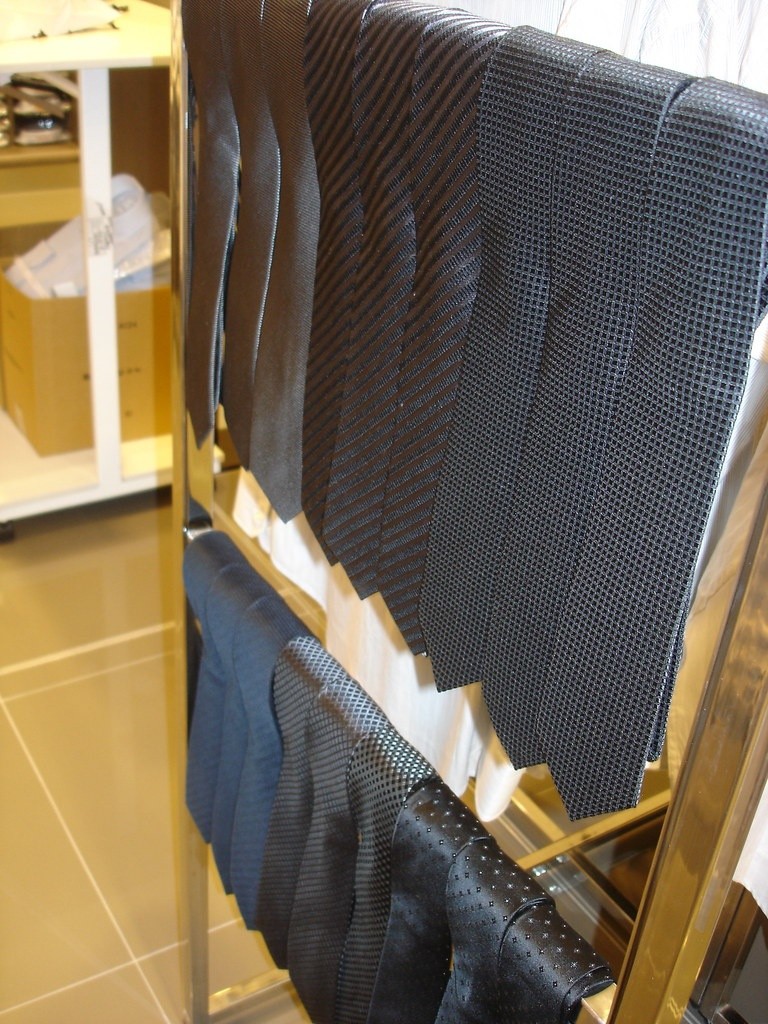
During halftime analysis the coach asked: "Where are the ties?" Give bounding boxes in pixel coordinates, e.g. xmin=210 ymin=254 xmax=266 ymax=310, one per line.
xmin=180 ymin=0 xmax=768 ymax=1024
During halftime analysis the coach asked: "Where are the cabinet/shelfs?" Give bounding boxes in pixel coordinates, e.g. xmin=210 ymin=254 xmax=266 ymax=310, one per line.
xmin=0 ymin=4 xmax=171 ymax=543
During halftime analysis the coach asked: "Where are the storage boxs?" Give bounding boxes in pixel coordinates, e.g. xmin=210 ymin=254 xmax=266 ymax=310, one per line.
xmin=0 ymin=254 xmax=174 ymax=459
